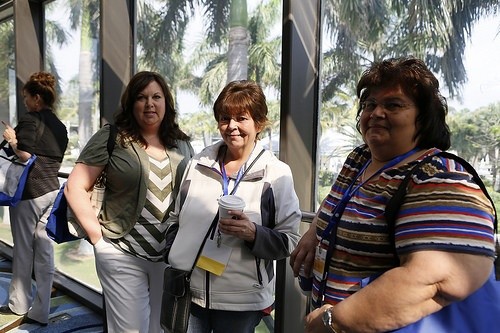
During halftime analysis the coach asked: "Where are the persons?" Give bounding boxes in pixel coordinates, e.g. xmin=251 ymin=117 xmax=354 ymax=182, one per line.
xmin=164 ymin=79 xmax=303 ymax=333
xmin=64 ymin=71 xmax=196 ymax=333
xmin=0 ymin=72 xmax=70 ymax=326
xmin=289 ymin=56 xmax=499 ymax=333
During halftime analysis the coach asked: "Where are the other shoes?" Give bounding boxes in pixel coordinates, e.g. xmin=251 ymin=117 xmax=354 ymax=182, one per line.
xmin=0 ymin=306 xmax=18 ymax=315
xmin=23 ymin=315 xmax=48 ymax=327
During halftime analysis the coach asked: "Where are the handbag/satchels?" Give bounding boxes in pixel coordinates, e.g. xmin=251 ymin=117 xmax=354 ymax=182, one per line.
xmin=160 ymin=266 xmax=192 ymax=333
xmin=45 ymin=121 xmax=119 ymax=245
xmin=0 ymin=110 xmax=44 ymax=208
xmin=359 ymin=152 xmax=500 ymax=333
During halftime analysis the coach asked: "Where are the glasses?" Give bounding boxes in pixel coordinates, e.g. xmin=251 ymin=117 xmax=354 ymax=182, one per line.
xmin=360 ymin=98 xmax=416 ymax=114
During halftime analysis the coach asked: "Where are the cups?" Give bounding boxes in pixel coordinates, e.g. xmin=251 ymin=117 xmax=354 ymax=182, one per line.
xmin=218 ymin=194 xmax=246 ymax=235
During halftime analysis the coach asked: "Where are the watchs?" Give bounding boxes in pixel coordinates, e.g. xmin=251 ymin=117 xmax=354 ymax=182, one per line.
xmin=322 ymin=306 xmax=342 ymax=333
